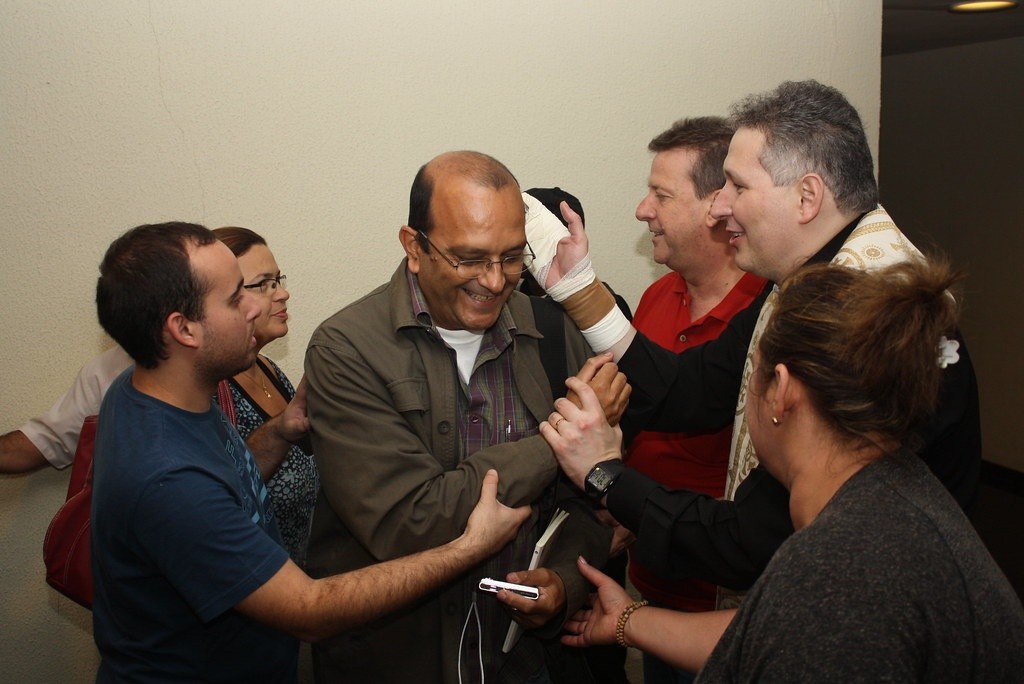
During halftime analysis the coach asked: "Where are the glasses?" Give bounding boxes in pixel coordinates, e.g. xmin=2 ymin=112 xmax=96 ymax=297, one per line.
xmin=244 ymin=275 xmax=288 ymax=295
xmin=417 ymin=227 xmax=536 ymax=280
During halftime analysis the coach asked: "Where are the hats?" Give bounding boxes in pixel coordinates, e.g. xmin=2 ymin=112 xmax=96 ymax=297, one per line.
xmin=522 ymin=186 xmax=585 ymax=230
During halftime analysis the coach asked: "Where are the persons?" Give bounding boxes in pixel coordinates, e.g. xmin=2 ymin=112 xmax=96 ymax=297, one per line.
xmin=213 ymin=226 xmax=318 ymax=563
xmin=596 ymin=117 xmax=769 ymax=684
xmin=0 ymin=344 xmax=136 ymax=474
xmin=520 ymin=81 xmax=986 ymax=610
xmin=526 ymin=187 xmax=633 ymax=324
xmin=305 ymin=151 xmax=632 ymax=684
xmin=91 ymin=221 xmax=531 ymax=684
xmin=561 ymin=259 xmax=1024 ymax=684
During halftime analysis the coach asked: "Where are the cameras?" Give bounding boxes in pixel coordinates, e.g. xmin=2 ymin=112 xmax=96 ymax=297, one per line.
xmin=479 ymin=578 xmax=540 ymax=599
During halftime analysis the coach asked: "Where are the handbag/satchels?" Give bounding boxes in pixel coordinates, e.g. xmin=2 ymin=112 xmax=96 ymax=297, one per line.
xmin=43 ymin=379 xmax=237 ymax=613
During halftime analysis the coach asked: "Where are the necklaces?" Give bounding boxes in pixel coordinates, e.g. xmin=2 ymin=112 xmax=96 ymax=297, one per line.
xmin=242 ymin=371 xmax=271 ymax=398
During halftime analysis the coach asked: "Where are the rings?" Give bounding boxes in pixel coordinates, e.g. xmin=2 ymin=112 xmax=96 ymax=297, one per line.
xmin=584 ymin=623 xmax=588 ymax=631
xmin=556 ymin=418 xmax=566 ymax=431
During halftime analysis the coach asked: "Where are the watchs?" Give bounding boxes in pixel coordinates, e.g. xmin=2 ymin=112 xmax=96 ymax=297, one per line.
xmin=584 ymin=457 xmax=628 ymax=507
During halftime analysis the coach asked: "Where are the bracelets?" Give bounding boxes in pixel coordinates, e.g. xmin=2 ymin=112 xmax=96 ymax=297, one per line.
xmin=617 ymin=600 xmax=650 ymax=647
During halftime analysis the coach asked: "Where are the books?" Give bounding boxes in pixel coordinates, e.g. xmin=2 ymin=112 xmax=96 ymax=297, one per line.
xmin=502 ymin=508 xmax=569 ymax=653
